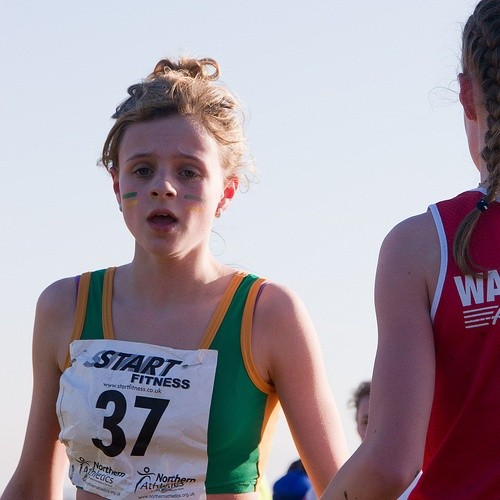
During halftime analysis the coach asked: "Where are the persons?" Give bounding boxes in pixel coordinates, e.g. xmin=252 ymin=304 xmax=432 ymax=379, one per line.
xmin=1 ymin=58 xmax=345 ymax=500
xmin=317 ymin=1 xmax=500 ymax=500
xmin=273 ymin=459 xmax=313 ymax=500
xmin=354 ymin=382 xmax=424 ymax=500
